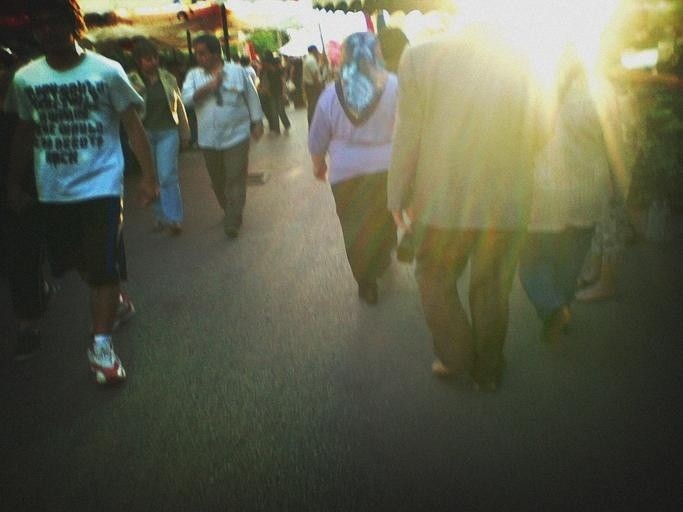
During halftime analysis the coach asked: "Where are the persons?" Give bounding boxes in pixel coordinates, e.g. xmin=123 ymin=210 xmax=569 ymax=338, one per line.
xmin=124 ymin=47 xmax=191 ymax=230
xmin=182 ymin=35 xmax=263 ymax=237
xmin=387 ymin=3 xmax=682 ymax=392
xmin=309 ymin=33 xmax=398 ymax=303
xmin=233 ymin=45 xmax=323 ymax=134
xmin=1 ymin=0 xmax=160 ymax=383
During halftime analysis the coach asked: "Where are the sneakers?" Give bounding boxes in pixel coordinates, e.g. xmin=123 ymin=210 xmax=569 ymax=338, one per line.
xmin=114 ymin=291 xmax=135 ymax=322
xmin=16 ymin=280 xmax=57 ymax=360
xmin=86 ymin=334 xmax=136 ymax=384
